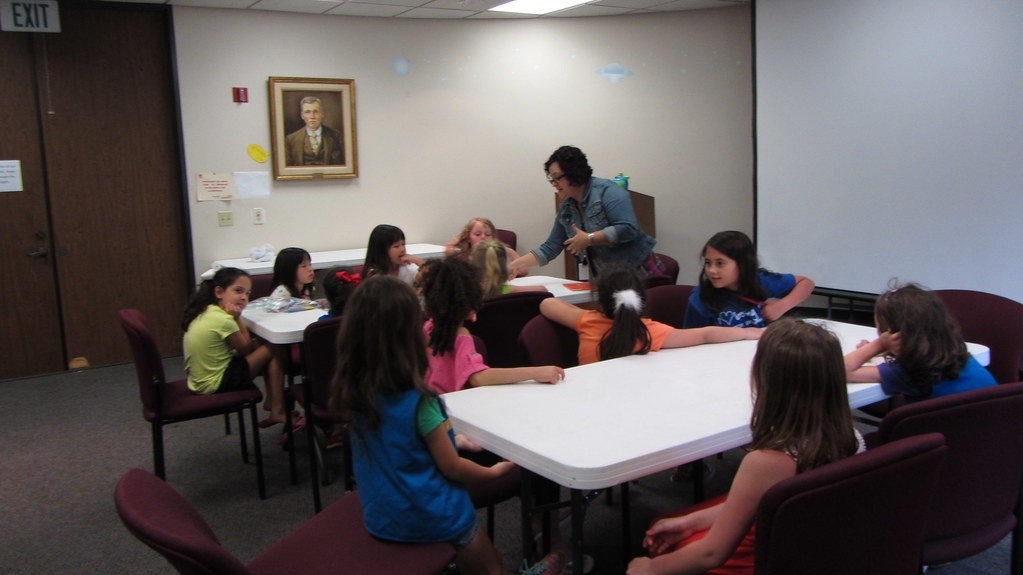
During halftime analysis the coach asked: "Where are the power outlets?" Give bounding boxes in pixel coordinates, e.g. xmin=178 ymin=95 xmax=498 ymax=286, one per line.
xmin=218 ymin=211 xmax=234 ymax=226
xmin=253 ymin=207 xmax=265 ymax=225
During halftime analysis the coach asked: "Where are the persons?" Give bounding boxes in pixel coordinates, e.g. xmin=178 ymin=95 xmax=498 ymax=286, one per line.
xmin=329 ymin=277 xmax=567 ymax=575
xmin=842 ymin=282 xmax=999 ymax=407
xmin=685 ymin=230 xmax=815 ymax=327
xmin=268 ymin=218 xmax=529 ymax=308
xmin=627 ymin=318 xmax=864 ymax=575
xmin=419 ymin=258 xmax=595 ymax=575
xmin=540 ymin=266 xmax=762 ymax=486
xmin=508 ymin=145 xmax=670 ymax=283
xmin=183 ymin=268 xmax=306 ymax=445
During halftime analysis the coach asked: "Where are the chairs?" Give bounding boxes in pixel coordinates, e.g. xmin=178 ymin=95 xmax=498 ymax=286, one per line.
xmin=108 ymin=229 xmax=1023 ymax=575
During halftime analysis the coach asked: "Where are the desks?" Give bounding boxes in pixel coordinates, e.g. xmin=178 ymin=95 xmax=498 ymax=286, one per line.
xmin=214 ymin=242 xmax=461 ymax=277
xmin=238 ymin=273 xmax=598 ymax=451
xmin=439 ymin=317 xmax=896 ymax=575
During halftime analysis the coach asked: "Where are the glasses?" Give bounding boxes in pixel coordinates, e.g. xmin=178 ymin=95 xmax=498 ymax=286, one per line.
xmin=548 ymin=173 xmax=566 ymax=184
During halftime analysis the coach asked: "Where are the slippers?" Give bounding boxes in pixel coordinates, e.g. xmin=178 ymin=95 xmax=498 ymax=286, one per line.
xmin=276 ymin=416 xmax=306 ymax=446
xmin=259 ymin=410 xmax=298 ymax=428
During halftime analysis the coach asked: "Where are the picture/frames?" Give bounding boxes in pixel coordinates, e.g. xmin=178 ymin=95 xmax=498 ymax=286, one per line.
xmin=268 ymin=76 xmax=359 ymax=181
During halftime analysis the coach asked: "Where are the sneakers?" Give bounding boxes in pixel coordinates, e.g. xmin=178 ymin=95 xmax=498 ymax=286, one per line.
xmin=518 ymin=551 xmax=567 ymax=575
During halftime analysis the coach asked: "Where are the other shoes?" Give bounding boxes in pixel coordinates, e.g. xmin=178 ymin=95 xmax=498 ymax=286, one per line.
xmin=564 ymin=554 xmax=595 ymax=574
xmin=326 ymin=433 xmax=344 ymax=449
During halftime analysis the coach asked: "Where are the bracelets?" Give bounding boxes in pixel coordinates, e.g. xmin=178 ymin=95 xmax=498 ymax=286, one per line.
xmin=588 ymin=232 xmax=594 ymax=246
